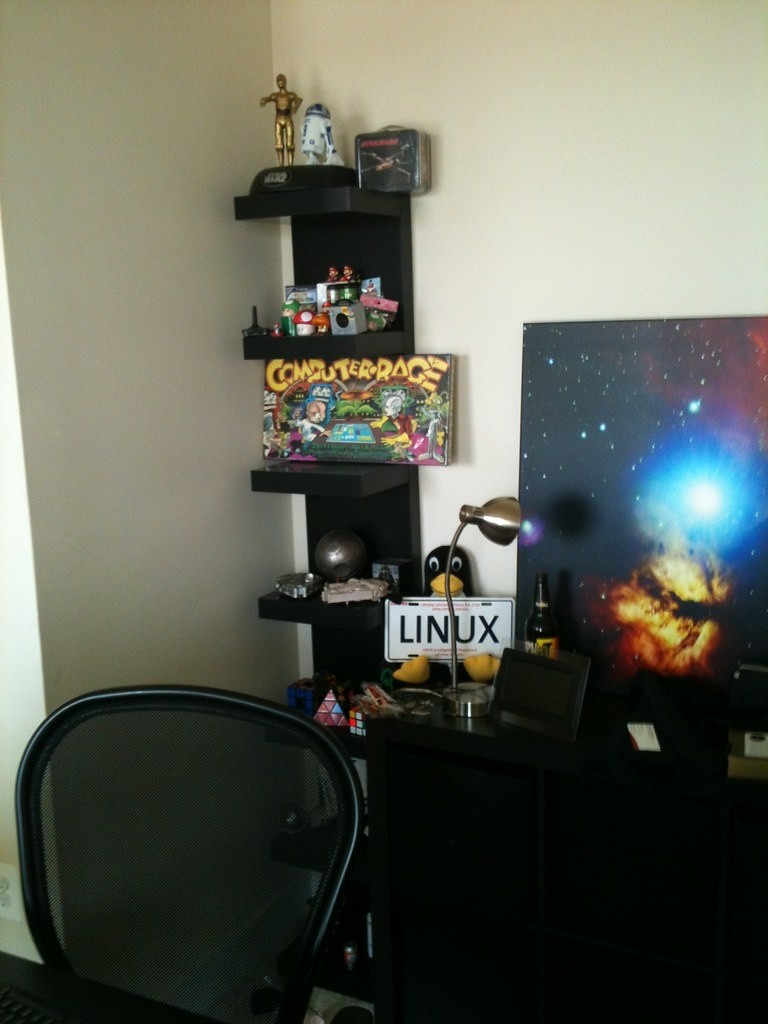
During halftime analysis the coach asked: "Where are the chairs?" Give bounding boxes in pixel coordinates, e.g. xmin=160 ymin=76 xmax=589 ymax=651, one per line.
xmin=12 ymin=684 xmax=365 ymax=1024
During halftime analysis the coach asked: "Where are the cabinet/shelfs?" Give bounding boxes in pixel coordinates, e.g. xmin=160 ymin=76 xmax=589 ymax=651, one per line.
xmin=374 ymin=670 xmax=768 ymax=1024
xmin=223 ymin=165 xmax=429 ymax=691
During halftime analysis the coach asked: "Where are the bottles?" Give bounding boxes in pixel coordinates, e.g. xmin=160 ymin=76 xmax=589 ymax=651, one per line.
xmin=525 ymin=572 xmax=559 ymax=662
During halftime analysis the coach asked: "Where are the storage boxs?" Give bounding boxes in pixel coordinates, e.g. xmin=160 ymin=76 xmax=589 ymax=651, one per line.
xmin=355 ymin=125 xmax=431 ymax=196
xmin=262 ymin=353 xmax=456 ymax=468
xmin=727 ymin=736 xmax=768 ymax=780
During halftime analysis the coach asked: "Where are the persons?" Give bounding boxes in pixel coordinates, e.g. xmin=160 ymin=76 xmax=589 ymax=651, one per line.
xmin=326 ymin=266 xmax=352 ymax=282
xmin=259 ymin=74 xmax=303 ymax=167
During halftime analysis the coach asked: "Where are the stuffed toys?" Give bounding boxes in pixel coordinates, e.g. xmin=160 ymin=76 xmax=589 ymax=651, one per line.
xmin=392 ymin=545 xmax=502 ymax=683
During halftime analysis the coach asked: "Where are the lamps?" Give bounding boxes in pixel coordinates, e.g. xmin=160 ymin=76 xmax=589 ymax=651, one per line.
xmin=441 ymin=495 xmax=521 ymax=719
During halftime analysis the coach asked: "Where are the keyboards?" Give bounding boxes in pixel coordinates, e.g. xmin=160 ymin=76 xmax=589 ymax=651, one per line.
xmin=0 ymin=982 xmax=78 ymax=1024
